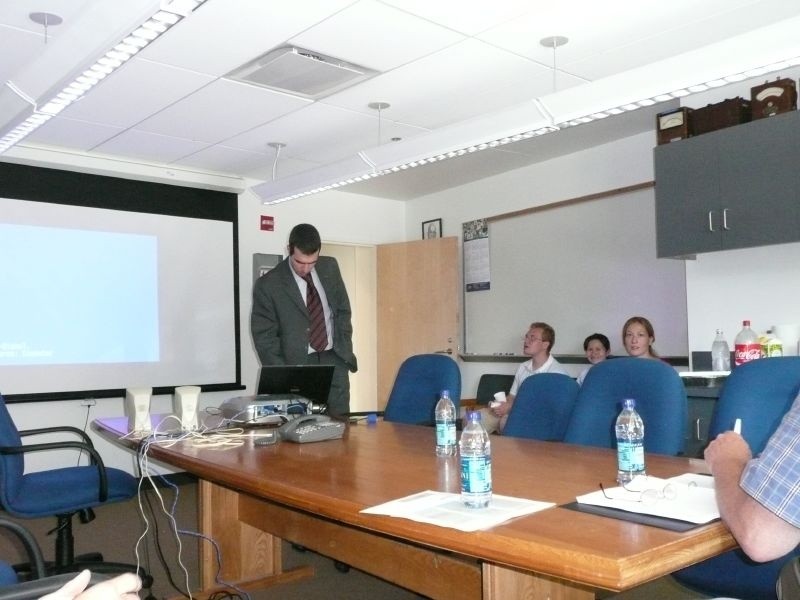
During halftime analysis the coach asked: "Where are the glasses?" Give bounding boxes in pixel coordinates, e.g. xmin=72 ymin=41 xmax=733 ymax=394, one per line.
xmin=525 ymin=335 xmax=544 ymax=342
xmin=600 ymin=480 xmax=676 ymax=505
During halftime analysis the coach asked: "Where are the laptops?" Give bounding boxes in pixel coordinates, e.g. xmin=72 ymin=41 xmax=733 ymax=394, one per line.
xmin=257 ymin=365 xmax=335 ymax=407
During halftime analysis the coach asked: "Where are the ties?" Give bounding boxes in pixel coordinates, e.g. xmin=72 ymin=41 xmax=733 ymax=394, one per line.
xmin=301 ymin=271 xmax=329 ymax=353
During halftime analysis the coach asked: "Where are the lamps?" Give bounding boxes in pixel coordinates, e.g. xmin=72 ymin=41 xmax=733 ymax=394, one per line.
xmin=0 ymin=0 xmax=208 ymax=162
xmin=247 ymin=15 xmax=800 ymax=207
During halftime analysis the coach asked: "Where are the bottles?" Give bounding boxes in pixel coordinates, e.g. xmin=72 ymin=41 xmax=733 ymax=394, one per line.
xmin=735 ymin=321 xmax=783 ymax=366
xmin=459 ymin=412 xmax=492 ymax=507
xmin=435 ymin=390 xmax=457 ymax=458
xmin=615 ymin=398 xmax=645 ymax=486
xmin=712 ymin=330 xmax=730 ymax=371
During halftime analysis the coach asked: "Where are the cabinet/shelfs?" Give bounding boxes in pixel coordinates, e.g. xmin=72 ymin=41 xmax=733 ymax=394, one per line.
xmin=680 ymin=370 xmax=731 ymax=459
xmin=652 ymin=110 xmax=800 ymax=260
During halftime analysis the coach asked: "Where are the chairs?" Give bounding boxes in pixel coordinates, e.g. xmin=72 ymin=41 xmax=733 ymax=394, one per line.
xmin=0 ymin=393 xmax=154 ymax=600
xmin=383 ymin=351 xmax=800 ymax=600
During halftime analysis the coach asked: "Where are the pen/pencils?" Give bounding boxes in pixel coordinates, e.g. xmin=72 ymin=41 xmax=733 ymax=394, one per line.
xmin=734 ymin=418 xmax=742 ymax=434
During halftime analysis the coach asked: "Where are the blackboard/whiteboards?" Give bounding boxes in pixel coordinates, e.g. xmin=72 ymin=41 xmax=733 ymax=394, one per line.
xmin=459 ymin=181 xmax=690 ymax=366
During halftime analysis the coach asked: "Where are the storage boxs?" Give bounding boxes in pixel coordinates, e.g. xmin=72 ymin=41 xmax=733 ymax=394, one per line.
xmin=687 ymin=97 xmax=751 ymax=137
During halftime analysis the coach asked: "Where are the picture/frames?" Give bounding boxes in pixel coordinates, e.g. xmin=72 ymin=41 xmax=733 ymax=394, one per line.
xmin=422 ymin=218 xmax=443 ymax=239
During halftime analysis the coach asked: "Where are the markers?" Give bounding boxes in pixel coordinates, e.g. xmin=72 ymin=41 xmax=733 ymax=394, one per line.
xmin=465 ymin=352 xmax=478 ymax=355
xmin=492 ymin=352 xmax=515 ymax=355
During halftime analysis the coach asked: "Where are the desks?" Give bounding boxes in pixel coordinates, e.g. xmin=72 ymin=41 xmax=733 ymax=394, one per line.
xmin=90 ymin=407 xmax=739 ymax=600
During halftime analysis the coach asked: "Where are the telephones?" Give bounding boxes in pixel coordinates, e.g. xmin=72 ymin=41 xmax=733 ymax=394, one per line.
xmin=279 ymin=414 xmax=347 ymax=442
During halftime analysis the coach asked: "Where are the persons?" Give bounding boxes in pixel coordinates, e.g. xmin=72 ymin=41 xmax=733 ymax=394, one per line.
xmin=576 ymin=334 xmax=611 ymax=386
xmin=478 ymin=323 xmax=568 ymax=436
xmin=704 ymin=388 xmax=800 ymax=563
xmin=622 ymin=317 xmax=656 ymax=358
xmin=251 ymin=224 xmax=357 ymax=413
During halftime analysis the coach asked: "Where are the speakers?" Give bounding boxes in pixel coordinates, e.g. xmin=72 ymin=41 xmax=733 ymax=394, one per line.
xmin=126 ymin=384 xmax=153 ymax=434
xmin=174 ymin=387 xmax=202 ymax=432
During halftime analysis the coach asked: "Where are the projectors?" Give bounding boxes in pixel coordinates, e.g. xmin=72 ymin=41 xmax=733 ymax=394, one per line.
xmin=219 ymin=394 xmax=313 ymax=427
